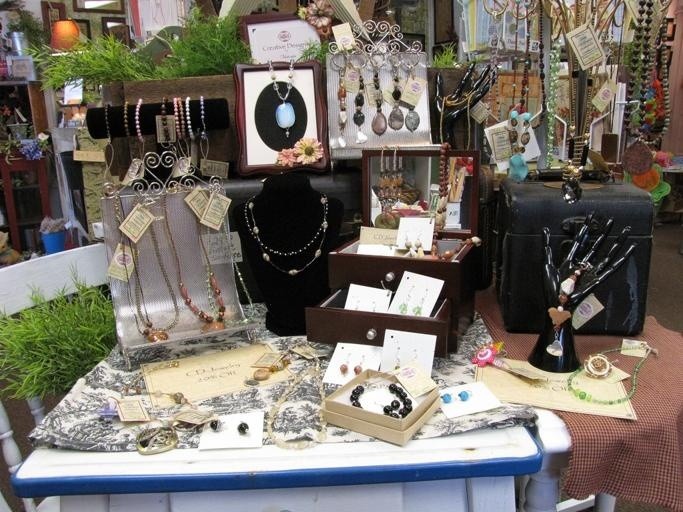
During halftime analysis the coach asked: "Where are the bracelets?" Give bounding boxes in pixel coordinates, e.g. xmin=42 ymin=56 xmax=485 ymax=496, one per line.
xmin=350 ymin=382 xmax=413 ymax=420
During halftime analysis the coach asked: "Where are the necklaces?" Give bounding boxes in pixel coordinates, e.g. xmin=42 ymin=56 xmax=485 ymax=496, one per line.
xmin=112 ymin=187 xmax=327 ymax=343
xmin=120 ymin=359 xmax=201 ymax=456
xmin=268 ymin=59 xmax=296 ymax=139
xmin=254 ymin=342 xmax=327 ymax=452
xmin=104 ymin=95 xmax=207 ymax=144
xmin=431 ymin=143 xmax=483 ymax=262
xmin=567 ymin=343 xmax=658 ymax=406
xmin=337 ymin=63 xmax=420 ymax=148
xmin=508 ymin=0 xmax=674 ymax=168
xmin=375 ymin=146 xmax=405 ymax=228
xmin=439 ymin=93 xmax=471 ymax=150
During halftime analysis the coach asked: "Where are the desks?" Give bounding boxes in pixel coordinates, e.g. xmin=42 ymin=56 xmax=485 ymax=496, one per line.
xmin=473 ymin=288 xmax=681 ymax=510
xmin=9 ymin=341 xmax=542 ymax=512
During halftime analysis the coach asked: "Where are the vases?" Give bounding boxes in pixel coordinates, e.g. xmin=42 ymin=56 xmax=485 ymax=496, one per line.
xmin=41 ymin=230 xmax=67 ymax=254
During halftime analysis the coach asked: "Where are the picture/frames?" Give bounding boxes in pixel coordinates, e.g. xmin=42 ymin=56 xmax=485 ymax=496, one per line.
xmin=5 ymin=55 xmax=36 ymax=81
xmin=231 ymin=9 xmax=329 ymax=178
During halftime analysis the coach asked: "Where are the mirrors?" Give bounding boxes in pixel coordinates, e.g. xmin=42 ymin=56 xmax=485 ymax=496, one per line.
xmin=361 ymin=148 xmax=479 ymax=240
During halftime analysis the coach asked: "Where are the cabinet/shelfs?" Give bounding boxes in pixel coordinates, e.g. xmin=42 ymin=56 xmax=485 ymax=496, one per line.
xmin=0 ymin=156 xmax=52 ymax=256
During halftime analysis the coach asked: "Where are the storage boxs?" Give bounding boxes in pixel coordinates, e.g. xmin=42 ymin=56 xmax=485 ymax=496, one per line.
xmin=495 ymin=178 xmax=655 ymax=337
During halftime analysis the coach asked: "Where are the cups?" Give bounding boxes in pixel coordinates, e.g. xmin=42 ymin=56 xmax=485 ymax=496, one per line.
xmin=8 ymin=123 xmax=29 ymax=139
